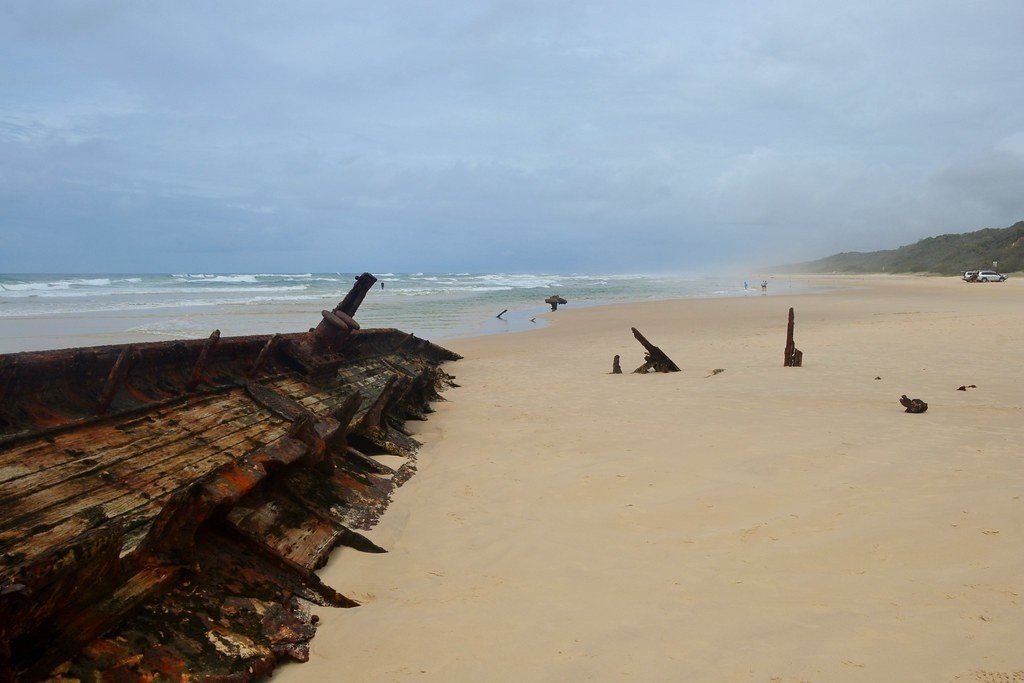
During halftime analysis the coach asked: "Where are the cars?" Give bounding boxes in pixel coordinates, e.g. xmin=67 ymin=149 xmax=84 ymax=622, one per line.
xmin=964 ymin=271 xmax=977 ymax=280
xmin=977 ymin=271 xmax=1008 ymax=282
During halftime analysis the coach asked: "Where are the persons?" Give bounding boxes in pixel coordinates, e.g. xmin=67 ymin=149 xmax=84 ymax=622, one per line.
xmin=381 ymin=281 xmax=384 ymax=290
xmin=761 ymin=281 xmax=768 ymax=288
xmin=744 ymin=281 xmax=747 ymax=289
